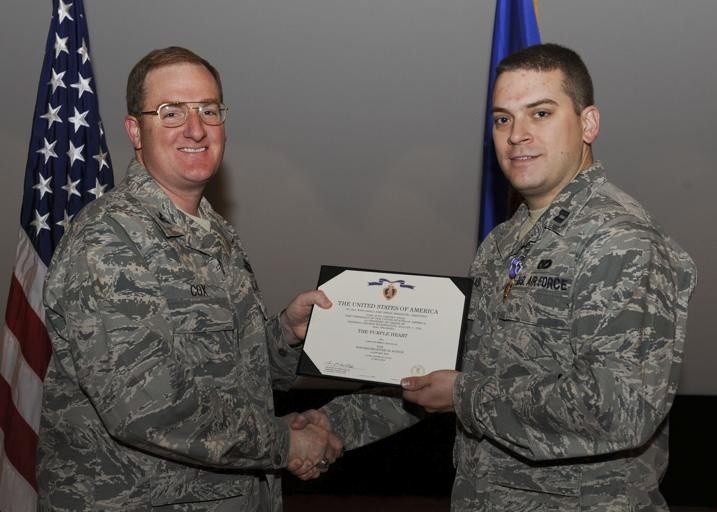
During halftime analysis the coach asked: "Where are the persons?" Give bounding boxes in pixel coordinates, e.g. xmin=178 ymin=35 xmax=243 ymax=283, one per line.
xmin=33 ymin=46 xmax=344 ymax=512
xmin=287 ymin=44 xmax=697 ymax=512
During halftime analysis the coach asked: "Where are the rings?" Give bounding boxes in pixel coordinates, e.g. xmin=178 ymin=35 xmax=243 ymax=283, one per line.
xmin=317 ymin=458 xmax=331 ymax=470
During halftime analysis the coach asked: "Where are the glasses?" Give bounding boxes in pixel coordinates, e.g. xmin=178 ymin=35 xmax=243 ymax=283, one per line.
xmin=136 ymin=102 xmax=232 ymax=128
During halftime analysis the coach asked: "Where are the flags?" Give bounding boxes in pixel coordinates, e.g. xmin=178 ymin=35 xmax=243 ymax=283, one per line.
xmin=480 ymin=1 xmax=542 ymax=249
xmin=0 ymin=0 xmax=113 ymax=511
